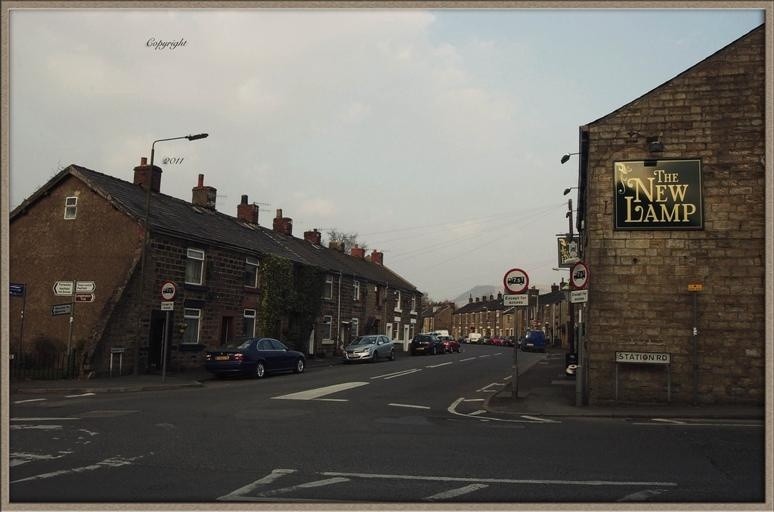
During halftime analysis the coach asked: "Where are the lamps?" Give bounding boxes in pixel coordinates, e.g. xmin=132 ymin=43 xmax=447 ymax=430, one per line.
xmin=559 ymin=150 xmax=582 ymax=163
xmin=562 ymin=185 xmax=581 ymax=195
xmin=566 ymin=207 xmax=579 ymax=220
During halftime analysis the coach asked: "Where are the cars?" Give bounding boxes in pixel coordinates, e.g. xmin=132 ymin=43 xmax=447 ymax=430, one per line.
xmin=342 ymin=334 xmax=395 ymax=363
xmin=411 ymin=329 xmax=545 ymax=358
xmin=201 ymin=336 xmax=307 ymax=382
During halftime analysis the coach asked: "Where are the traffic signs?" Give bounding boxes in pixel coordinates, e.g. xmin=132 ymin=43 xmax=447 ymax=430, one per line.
xmin=75 ymin=280 xmax=96 ymax=292
xmin=9 ymin=283 xmax=24 ymax=297
xmin=50 ymin=303 xmax=71 ymax=317
xmin=74 ymin=293 xmax=96 ymax=303
xmin=52 ymin=280 xmax=72 ymax=297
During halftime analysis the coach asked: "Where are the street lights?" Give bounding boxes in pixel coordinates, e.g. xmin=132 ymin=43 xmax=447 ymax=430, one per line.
xmin=482 ymin=307 xmax=488 ymax=321
xmin=133 ymin=131 xmax=210 ymax=377
xmin=532 ymin=294 xmax=539 ymax=320
xmin=552 ymin=267 xmax=570 ymax=273
